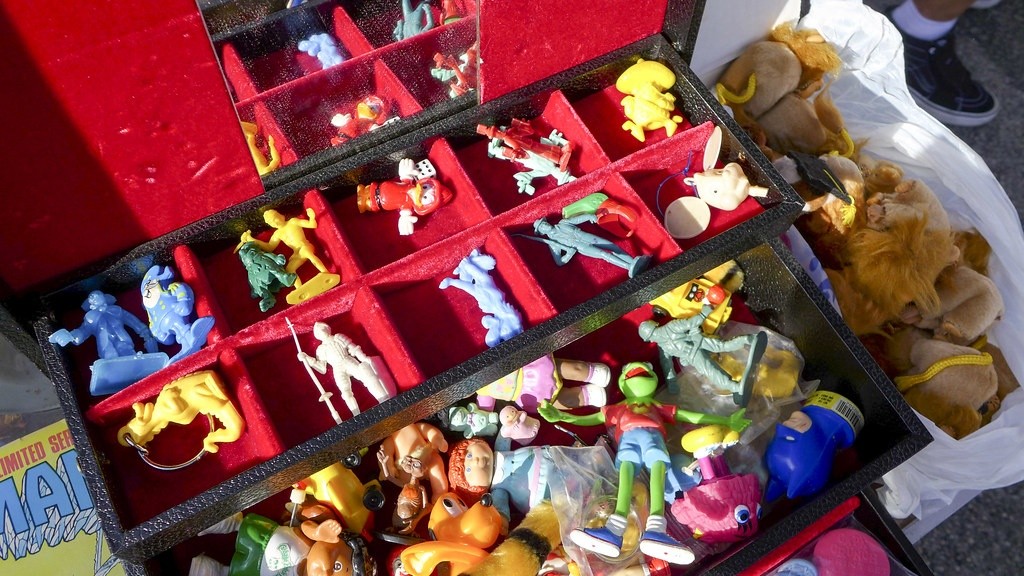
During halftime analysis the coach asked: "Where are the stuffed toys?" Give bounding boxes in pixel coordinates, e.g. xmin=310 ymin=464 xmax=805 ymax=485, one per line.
xmin=715 ymin=21 xmax=1021 ymax=440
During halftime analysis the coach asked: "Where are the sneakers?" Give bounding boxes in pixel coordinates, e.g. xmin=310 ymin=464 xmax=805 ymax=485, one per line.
xmin=883 ymin=5 xmax=1000 ymax=127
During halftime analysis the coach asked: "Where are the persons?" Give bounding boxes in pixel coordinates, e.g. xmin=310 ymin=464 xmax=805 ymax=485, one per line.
xmin=394 ymin=0 xmax=434 ymax=42
xmin=532 ymin=213 xmax=633 ymax=271
xmin=244 ymin=130 xmax=280 ymax=176
xmin=881 ymin=0 xmax=1001 ymax=127
xmin=241 ymin=208 xmax=326 ymax=289
xmin=189 ymin=303 xmax=768 ymax=576
xmin=438 ymin=258 xmax=525 ymax=348
xmin=48 ymin=290 xmax=159 ymax=359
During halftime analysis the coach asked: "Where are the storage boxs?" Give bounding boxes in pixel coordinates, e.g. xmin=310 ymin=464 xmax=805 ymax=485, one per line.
xmin=0 ymin=0 xmax=934 ymax=576
